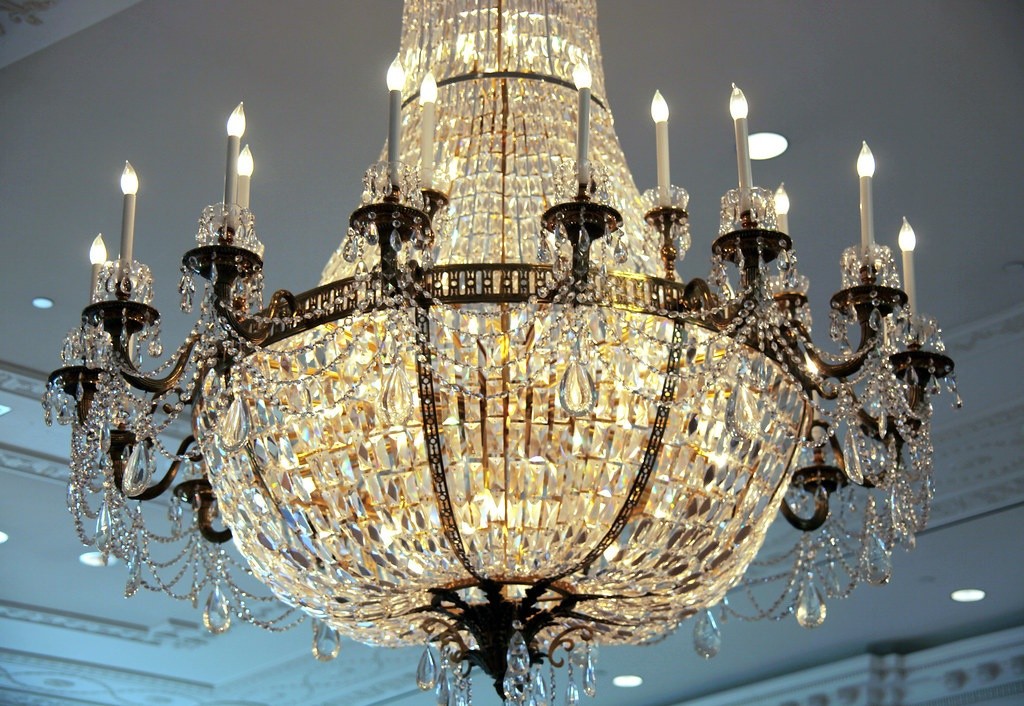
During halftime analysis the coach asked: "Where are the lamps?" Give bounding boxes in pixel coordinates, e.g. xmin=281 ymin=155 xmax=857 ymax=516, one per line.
xmin=42 ymin=0 xmax=963 ymax=705
